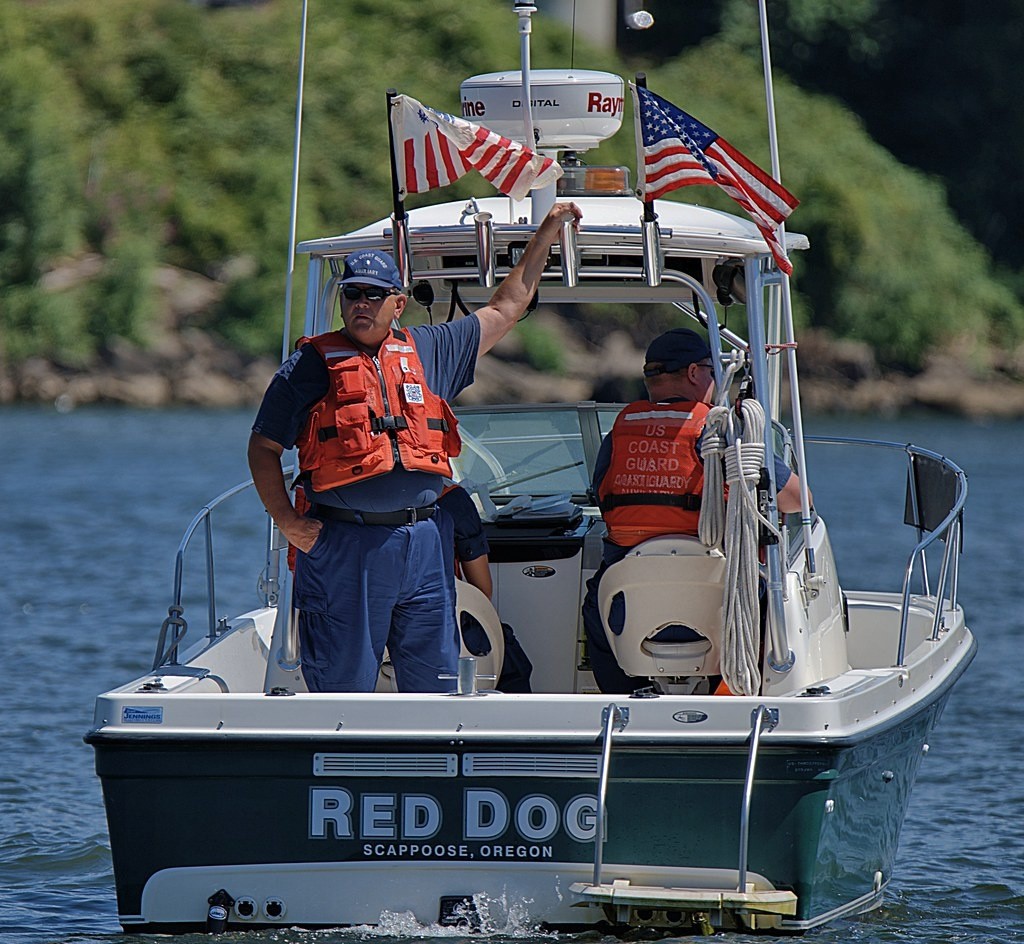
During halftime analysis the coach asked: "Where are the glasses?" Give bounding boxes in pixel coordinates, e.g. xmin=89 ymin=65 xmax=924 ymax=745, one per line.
xmin=342 ymin=286 xmax=392 ymax=301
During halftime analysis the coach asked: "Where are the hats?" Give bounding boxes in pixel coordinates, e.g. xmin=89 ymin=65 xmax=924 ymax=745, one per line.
xmin=338 ymin=248 xmax=403 ymax=291
xmin=643 ymin=327 xmax=711 ymax=378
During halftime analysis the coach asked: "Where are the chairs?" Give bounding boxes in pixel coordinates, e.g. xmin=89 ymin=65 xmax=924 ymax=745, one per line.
xmin=598 ymin=534 xmax=727 ymax=695
xmin=454 ymin=575 xmax=505 ymax=695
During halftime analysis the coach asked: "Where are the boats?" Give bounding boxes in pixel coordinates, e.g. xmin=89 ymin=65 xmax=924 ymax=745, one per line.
xmin=80 ymin=0 xmax=972 ymax=944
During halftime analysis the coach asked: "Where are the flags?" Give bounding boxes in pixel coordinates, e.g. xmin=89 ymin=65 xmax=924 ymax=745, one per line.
xmin=627 ymin=79 xmax=802 ymax=279
xmin=391 ymin=92 xmax=564 ymax=205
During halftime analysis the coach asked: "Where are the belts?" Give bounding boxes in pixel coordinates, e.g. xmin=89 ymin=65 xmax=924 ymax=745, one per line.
xmin=309 ymin=501 xmax=437 ymax=527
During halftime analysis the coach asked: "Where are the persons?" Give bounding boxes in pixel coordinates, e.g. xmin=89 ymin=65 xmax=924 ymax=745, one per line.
xmin=581 ymin=327 xmax=814 ymax=696
xmin=246 ymin=201 xmax=584 ymax=693
xmin=435 ymin=479 xmax=533 ymax=694
